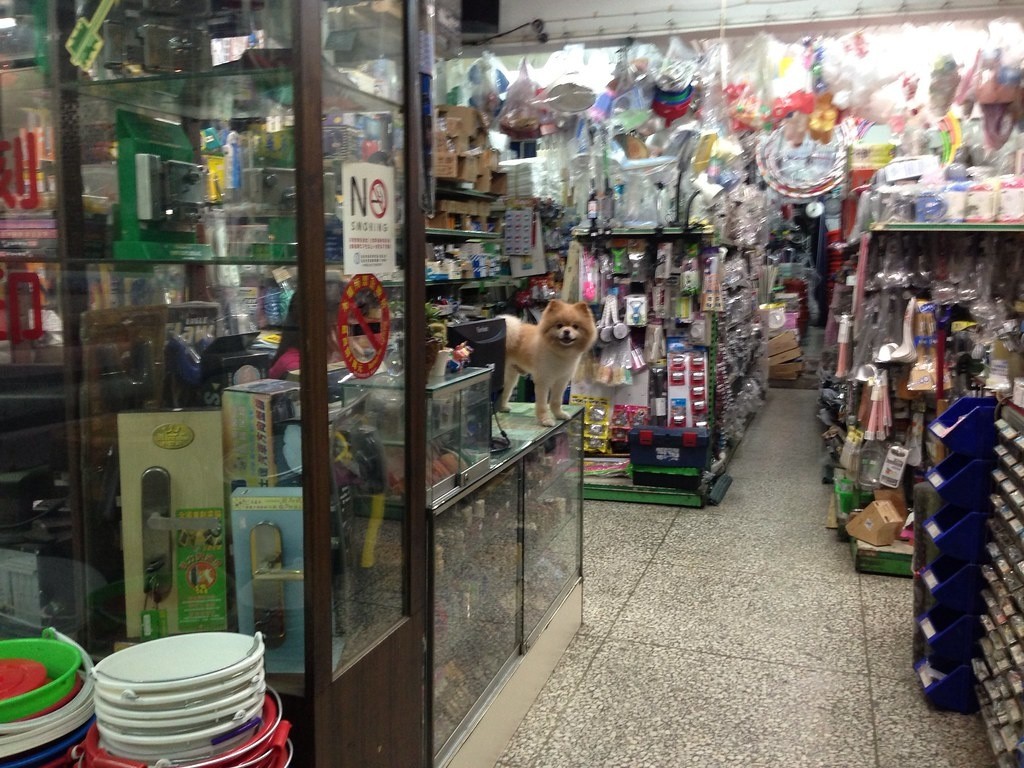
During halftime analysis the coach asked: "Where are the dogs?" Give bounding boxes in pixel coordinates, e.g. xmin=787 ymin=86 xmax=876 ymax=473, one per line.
xmin=493 ymin=299 xmax=598 ymax=428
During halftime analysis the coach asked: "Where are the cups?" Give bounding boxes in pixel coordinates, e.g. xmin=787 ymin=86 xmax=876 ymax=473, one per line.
xmin=909 ymin=163 xmax=1024 ymax=225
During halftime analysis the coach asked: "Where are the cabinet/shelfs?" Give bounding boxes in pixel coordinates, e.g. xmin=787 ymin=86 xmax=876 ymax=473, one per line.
xmin=561 ymin=203 xmax=773 ymax=511
xmin=424 ymin=179 xmax=512 ymax=380
xmin=55 ymin=0 xmax=436 ymax=768
xmin=966 ymin=394 xmax=1023 ymax=768
xmin=333 ymin=399 xmax=586 ymax=768
xmin=827 ymin=221 xmax=1024 ymax=584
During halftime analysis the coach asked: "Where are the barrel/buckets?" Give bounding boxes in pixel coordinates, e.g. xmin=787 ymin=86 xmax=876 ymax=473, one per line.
xmin=1 ymin=625 xmax=294 ymax=768
